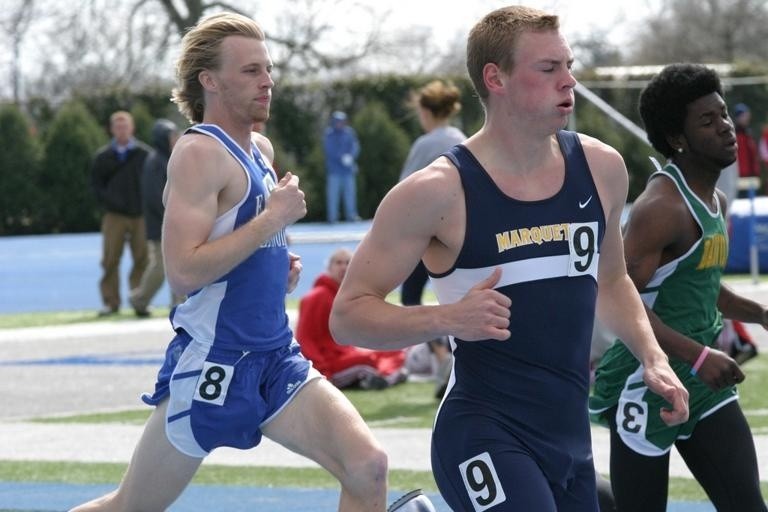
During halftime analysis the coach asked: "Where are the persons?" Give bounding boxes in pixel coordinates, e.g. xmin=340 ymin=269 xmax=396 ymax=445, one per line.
xmin=328 ymin=3 xmax=690 ymax=512
xmin=398 ymin=80 xmax=468 ymax=398
xmin=732 ymin=102 xmax=760 ymax=198
xmin=324 ymin=111 xmax=362 ymax=226
xmin=90 ymin=111 xmax=156 ymax=319
xmin=67 ymin=13 xmax=389 ymax=512
xmin=588 ymin=63 xmax=768 ymax=512
xmin=295 ymin=248 xmax=408 ymax=390
xmin=128 ymin=119 xmax=179 ymax=317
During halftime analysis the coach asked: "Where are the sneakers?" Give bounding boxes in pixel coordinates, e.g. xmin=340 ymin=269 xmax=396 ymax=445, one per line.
xmin=131 ymin=295 xmax=150 ymax=316
xmin=100 ymin=303 xmax=119 ymax=314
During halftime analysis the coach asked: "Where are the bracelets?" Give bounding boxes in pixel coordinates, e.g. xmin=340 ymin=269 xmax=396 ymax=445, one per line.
xmin=690 ymin=345 xmax=710 ymax=376
